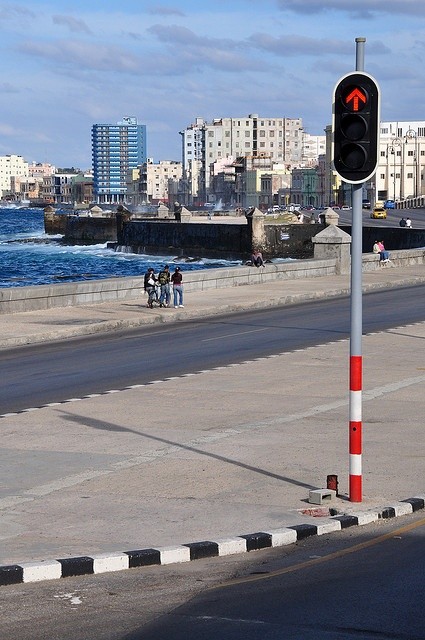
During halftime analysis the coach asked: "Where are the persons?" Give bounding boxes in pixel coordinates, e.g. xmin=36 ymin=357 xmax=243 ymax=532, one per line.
xmin=310 ymin=212 xmax=316 ymax=223
xmin=406 ymin=217 xmax=412 ymax=227
xmin=143 ymin=267 xmax=158 ymax=309
xmin=157 ymin=264 xmax=171 ymax=308
xmin=379 ymin=240 xmax=391 ymax=263
xmin=251 ymin=248 xmax=265 ymax=268
xmin=170 ymin=266 xmax=184 ymax=309
xmin=373 ymin=241 xmax=387 ymax=263
xmin=318 ymin=211 xmax=323 ymax=223
xmin=399 ymin=217 xmax=406 ymax=227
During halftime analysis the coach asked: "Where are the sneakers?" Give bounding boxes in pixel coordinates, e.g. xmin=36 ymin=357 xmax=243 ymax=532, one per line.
xmin=166 ymin=304 xmax=170 ymax=308
xmin=175 ymin=306 xmax=178 ymax=308
xmin=160 ymin=304 xmax=163 ymax=307
xmin=384 ymin=259 xmax=392 ymax=263
xmin=380 ymin=260 xmax=385 ymax=263
xmin=179 ymin=305 xmax=184 ymax=308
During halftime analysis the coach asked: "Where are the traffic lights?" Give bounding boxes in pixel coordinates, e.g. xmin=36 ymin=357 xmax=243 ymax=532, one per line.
xmin=330 ymin=71 xmax=382 ymax=185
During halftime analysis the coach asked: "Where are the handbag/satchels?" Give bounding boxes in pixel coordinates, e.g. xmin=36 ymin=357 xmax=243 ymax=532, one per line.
xmin=148 ymin=274 xmax=155 ymax=286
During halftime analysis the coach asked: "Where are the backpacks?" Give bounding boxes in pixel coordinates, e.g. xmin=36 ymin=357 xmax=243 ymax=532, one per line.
xmin=159 ymin=272 xmax=170 ymax=285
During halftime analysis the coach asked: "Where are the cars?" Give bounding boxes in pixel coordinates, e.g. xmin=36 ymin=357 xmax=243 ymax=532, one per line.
xmin=332 ymin=206 xmax=340 ymax=211
xmin=375 ymin=201 xmax=385 ymax=208
xmin=370 ymin=208 xmax=387 ymax=219
xmin=362 ymin=199 xmax=371 ymax=208
xmin=384 ymin=199 xmax=395 ymax=209
xmin=270 ymin=202 xmax=329 ymax=212
xmin=341 ymin=205 xmax=350 ymax=211
